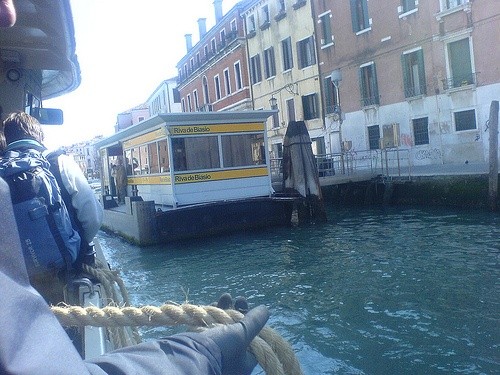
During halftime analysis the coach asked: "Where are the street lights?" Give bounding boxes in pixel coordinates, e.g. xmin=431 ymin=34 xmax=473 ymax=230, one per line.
xmin=329 ymin=68 xmax=345 ymax=175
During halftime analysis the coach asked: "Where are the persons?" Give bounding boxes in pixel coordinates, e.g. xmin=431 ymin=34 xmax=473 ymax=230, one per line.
xmin=126 ymin=157 xmax=139 ymax=174
xmin=111 ymin=158 xmax=127 ymax=205
xmin=0 ymin=177 xmax=270 ymax=375
xmin=0 ymin=111 xmax=104 ymax=356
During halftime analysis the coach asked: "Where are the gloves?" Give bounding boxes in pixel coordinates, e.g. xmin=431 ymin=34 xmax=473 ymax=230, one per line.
xmin=202 ymin=293 xmax=270 ymax=375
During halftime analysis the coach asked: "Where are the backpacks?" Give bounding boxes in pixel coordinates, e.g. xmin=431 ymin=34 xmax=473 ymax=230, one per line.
xmin=0 ymin=148 xmax=81 ymax=279
